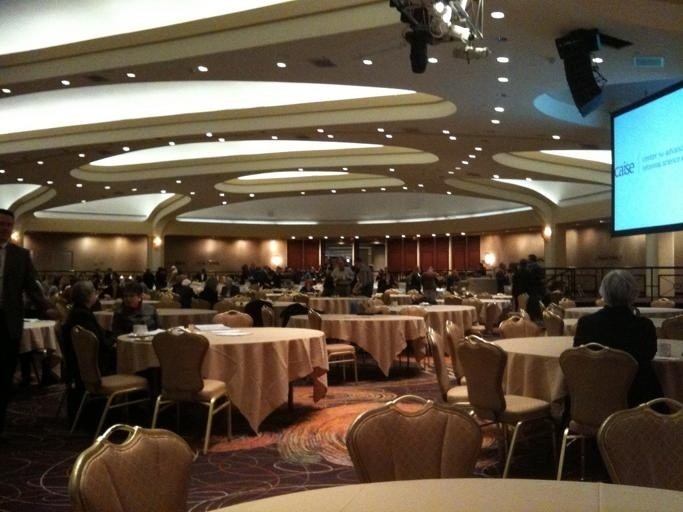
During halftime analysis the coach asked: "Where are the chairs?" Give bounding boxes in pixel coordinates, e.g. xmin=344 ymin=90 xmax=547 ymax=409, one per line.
xmin=505 ymin=293 xmax=529 ymax=320
xmin=598 ymin=397 xmax=682 ymax=492
xmin=539 ymin=301 xmax=546 ymax=312
xmin=211 ymin=309 xmax=252 ymax=327
xmin=54 ymin=322 xmax=80 ymax=426
xmin=497 ymin=316 xmax=539 ymax=339
xmin=293 ymin=294 xmax=310 ymax=307
xmin=64 ymin=325 xmax=150 ymax=442
xmin=542 ymin=310 xmax=564 ymax=336
xmin=343 ymin=393 xmax=482 ymax=484
xmin=278 ymin=293 xmax=292 ymax=302
xmin=559 ymin=297 xmax=576 ymax=308
xmin=150 ymin=327 xmax=233 ymax=455
xmin=426 ymin=326 xmax=510 ymax=477
xmin=457 ymin=335 xmax=549 ymax=477
xmin=550 ymin=303 xmax=565 ymax=318
xmin=556 ymin=344 xmax=638 ymax=483
xmin=68 ymin=425 xmax=193 ymax=512
xmin=191 ymin=296 xmax=210 ymax=309
xmin=260 ymin=303 xmax=276 ymax=327
xmin=308 ymin=309 xmax=359 ymax=388
xmin=651 ymin=298 xmax=675 ymax=309
xmin=445 ymin=296 xmax=462 ymax=305
xmin=662 ymin=316 xmax=683 ymax=339
xmin=444 ymin=319 xmax=467 ymax=386
xmin=398 ymin=308 xmax=429 ymax=373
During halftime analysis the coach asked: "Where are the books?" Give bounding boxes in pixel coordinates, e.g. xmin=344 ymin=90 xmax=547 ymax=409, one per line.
xmin=195 ymin=322 xmax=230 ymax=331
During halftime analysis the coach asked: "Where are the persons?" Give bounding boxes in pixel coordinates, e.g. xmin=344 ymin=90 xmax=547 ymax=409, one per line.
xmin=436 ymin=273 xmax=445 ymax=287
xmin=299 ymin=265 xmax=321 ymax=279
xmin=527 ymin=254 xmax=542 ymax=280
xmin=318 ymin=257 xmax=358 ymax=297
xmin=496 ymin=262 xmax=505 ymax=293
xmin=376 ymin=268 xmax=399 ymax=293
xmin=168 ymin=264 xmax=177 ymax=285
xmin=300 ymin=279 xmax=319 ymax=293
xmin=512 ymin=259 xmax=546 ymax=321
xmin=173 ymin=279 xmax=199 ymax=308
xmin=220 ymin=273 xmax=240 ymax=299
xmin=1 ymin=209 xmax=58 ymax=435
xmin=560 ymin=271 xmax=670 ymax=438
xmin=196 ymin=269 xmax=208 ymax=281
xmin=504 ymin=263 xmax=517 ymax=286
xmin=92 ymin=268 xmax=128 ymax=299
xmin=240 ymin=263 xmax=298 ymax=289
xmin=142 ymin=269 xmax=154 ymax=289
xmin=446 ymin=270 xmax=460 ymax=295
xmin=113 ymin=286 xmax=163 ymax=346
xmin=406 ymin=268 xmax=421 ymax=293
xmin=199 ymin=277 xmax=218 ymax=310
xmin=421 ymin=266 xmax=441 ymax=305
xmin=30 ymin=267 xmax=78 ymax=297
xmin=61 ymin=280 xmax=118 ymax=384
xmin=154 ymin=267 xmax=168 ymax=291
xmin=78 ymin=272 xmax=89 ymax=280
xmin=355 ymin=258 xmax=375 ymax=298
xmin=478 ymin=264 xmax=486 ymax=276
xmin=476 ymin=263 xmax=487 ymax=277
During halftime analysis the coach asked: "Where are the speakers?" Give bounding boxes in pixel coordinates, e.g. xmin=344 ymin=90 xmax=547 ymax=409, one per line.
xmin=556 ymin=29 xmax=606 ymax=116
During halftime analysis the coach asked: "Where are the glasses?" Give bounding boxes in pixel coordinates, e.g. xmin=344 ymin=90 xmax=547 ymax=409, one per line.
xmin=123 ymin=294 xmax=140 ymax=298
